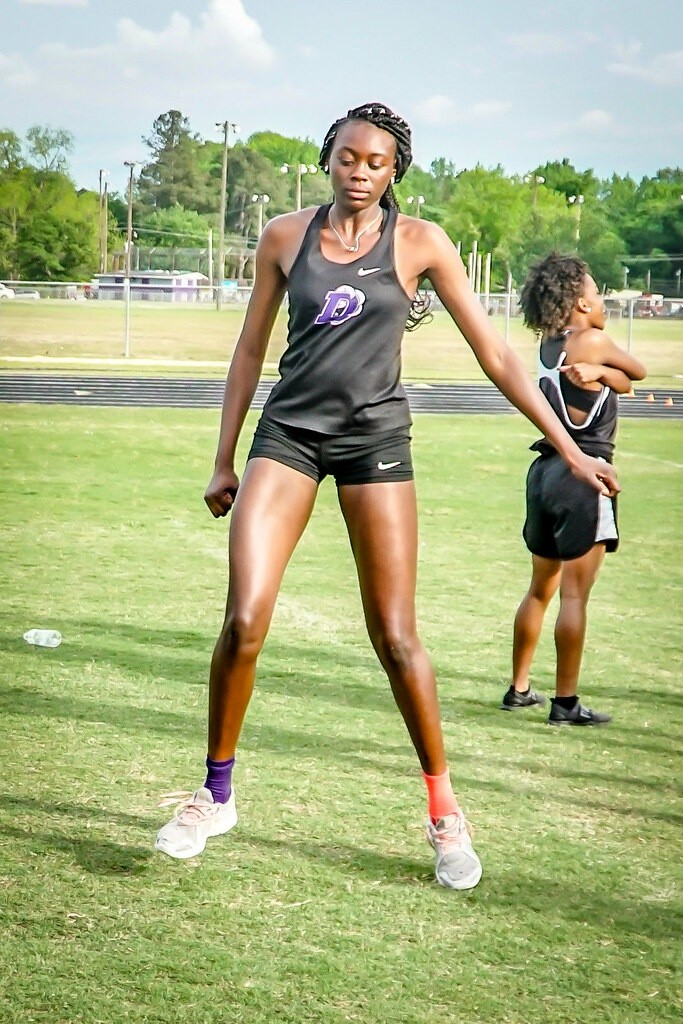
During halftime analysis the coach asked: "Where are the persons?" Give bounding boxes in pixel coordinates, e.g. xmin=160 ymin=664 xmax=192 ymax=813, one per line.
xmin=154 ymin=102 xmax=620 ymax=893
xmin=501 ymin=250 xmax=647 ymax=725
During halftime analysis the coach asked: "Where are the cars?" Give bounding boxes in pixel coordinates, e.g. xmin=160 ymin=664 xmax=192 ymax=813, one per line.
xmin=0 ymin=283 xmax=40 ymax=301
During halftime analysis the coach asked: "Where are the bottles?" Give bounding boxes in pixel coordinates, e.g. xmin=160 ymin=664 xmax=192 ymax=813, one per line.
xmin=23 ymin=629 xmax=62 ymax=647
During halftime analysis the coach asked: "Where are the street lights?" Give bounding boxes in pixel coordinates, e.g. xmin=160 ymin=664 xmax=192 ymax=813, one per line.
xmin=406 ymin=195 xmax=426 ymax=219
xmin=251 ymin=194 xmax=269 ymax=239
xmin=282 ymin=163 xmax=319 ymax=212
xmin=214 ymin=119 xmax=240 ymax=309
xmin=524 ymin=174 xmax=545 ymax=205
xmin=123 ymin=160 xmax=135 ymax=280
xmin=567 ymin=194 xmax=585 ymax=255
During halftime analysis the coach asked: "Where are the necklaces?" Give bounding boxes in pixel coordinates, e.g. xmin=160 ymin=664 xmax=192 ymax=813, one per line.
xmin=328 ymin=203 xmax=383 ymax=252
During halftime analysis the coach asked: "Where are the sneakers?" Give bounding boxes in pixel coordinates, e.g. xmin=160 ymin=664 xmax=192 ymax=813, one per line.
xmin=426 ymin=809 xmax=482 ymax=889
xmin=548 ymin=697 xmax=611 ymax=725
xmin=500 ymin=685 xmax=546 ymax=711
xmin=155 ymin=788 xmax=239 ymax=858
